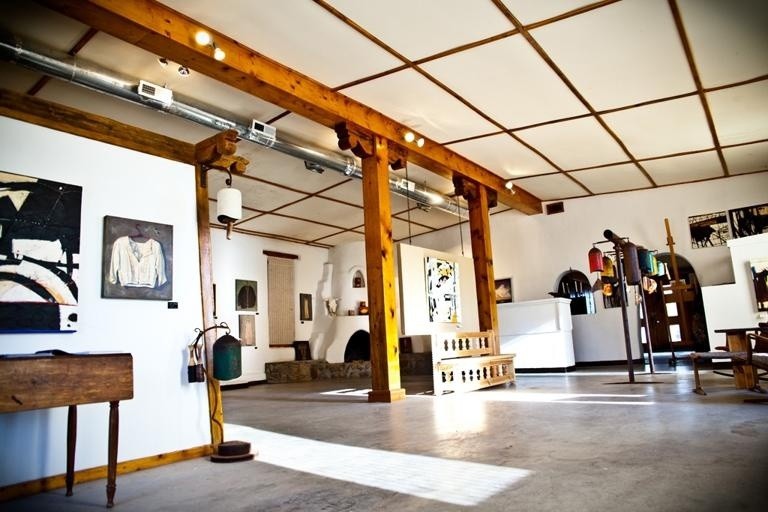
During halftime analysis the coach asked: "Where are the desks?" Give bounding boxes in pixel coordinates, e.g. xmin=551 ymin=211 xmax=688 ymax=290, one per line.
xmin=714 ymin=327 xmax=761 ymax=390
xmin=0 ymin=353 xmax=132 ymax=512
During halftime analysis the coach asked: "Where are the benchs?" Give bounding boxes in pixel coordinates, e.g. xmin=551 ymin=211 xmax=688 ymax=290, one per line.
xmin=689 ymin=352 xmax=767 ymax=396
xmin=427 ymin=329 xmax=516 ymax=396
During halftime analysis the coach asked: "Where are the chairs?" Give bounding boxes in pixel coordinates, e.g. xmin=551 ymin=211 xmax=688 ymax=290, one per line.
xmin=732 ymin=322 xmax=767 ymax=405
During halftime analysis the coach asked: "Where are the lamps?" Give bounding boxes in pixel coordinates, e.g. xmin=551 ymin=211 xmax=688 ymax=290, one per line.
xmin=187 ymin=321 xmax=254 ymax=461
xmin=200 ymin=165 xmax=243 ymax=240
xmin=589 ymin=229 xmax=678 ymax=384
